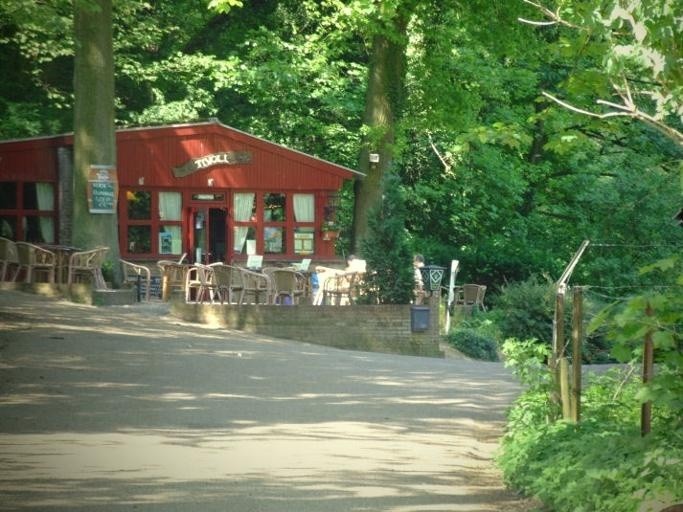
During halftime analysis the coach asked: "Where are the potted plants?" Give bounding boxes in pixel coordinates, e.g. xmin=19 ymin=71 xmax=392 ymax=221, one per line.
xmin=321 ymin=220 xmax=338 ymax=239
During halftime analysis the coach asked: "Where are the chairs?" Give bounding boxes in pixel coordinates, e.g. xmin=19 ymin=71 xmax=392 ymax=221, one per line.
xmin=439 ymin=283 xmax=487 ymax=313
xmin=2 ymin=237 xmax=21 ymax=282
xmin=118 ymin=258 xmax=159 ymax=304
xmin=68 ymin=245 xmax=112 ymax=291
xmin=156 ymin=259 xmax=377 ymax=310
xmin=12 ymin=242 xmax=56 ymax=284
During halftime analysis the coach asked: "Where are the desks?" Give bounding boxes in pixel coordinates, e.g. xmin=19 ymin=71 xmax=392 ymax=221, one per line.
xmin=40 ymin=244 xmax=81 ymax=283
